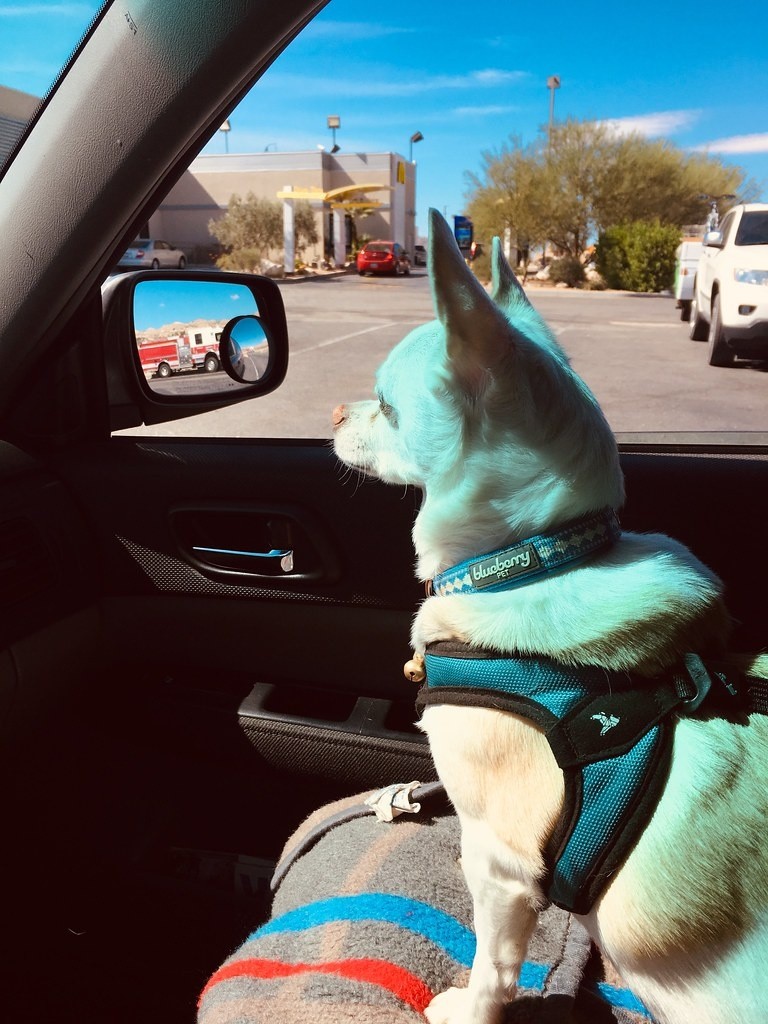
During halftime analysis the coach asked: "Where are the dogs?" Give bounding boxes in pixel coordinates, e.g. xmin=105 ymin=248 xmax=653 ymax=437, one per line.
xmin=329 ymin=206 xmax=767 ymax=1023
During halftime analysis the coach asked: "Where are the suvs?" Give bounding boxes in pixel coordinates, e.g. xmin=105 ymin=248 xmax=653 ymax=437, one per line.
xmin=689 ymin=201 xmax=768 ymax=369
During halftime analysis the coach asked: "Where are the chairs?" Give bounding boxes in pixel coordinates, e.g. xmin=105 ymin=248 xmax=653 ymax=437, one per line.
xmin=192 ymin=782 xmax=666 ymax=1022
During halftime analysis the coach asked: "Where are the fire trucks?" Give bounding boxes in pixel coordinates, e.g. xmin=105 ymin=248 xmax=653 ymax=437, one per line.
xmin=137 ymin=325 xmax=224 ymax=379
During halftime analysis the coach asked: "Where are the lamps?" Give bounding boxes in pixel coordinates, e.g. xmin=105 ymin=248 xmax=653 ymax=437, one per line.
xmin=328 ymin=115 xmax=341 ymax=154
xmin=409 ymin=131 xmax=423 ymax=163
xmin=219 ymin=117 xmax=231 ymax=154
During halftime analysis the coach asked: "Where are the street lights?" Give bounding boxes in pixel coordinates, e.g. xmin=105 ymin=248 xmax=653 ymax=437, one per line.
xmin=542 ymin=74 xmax=562 ymax=268
xmin=219 ymin=118 xmax=233 ymax=154
xmin=326 ymin=114 xmax=340 ymax=155
xmin=409 ymin=131 xmax=424 ymax=164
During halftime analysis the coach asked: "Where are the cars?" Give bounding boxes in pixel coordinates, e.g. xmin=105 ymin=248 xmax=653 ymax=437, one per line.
xmin=116 ymin=239 xmax=188 ymax=270
xmin=357 ymin=238 xmax=411 ymax=277
xmin=414 ymin=245 xmax=427 ymax=266
